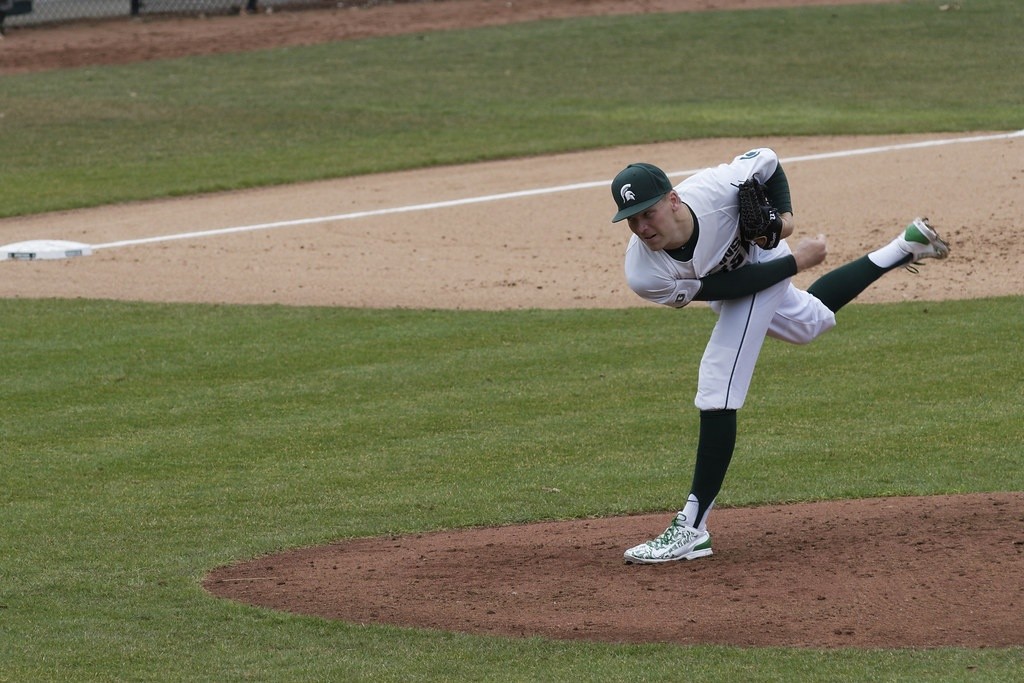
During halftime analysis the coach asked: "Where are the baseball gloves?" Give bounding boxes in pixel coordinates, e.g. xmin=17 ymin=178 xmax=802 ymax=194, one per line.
xmin=735 ymin=176 xmax=784 ymax=251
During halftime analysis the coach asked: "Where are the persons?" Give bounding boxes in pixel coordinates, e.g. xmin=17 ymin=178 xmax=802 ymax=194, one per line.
xmin=611 ymin=149 xmax=951 ymax=563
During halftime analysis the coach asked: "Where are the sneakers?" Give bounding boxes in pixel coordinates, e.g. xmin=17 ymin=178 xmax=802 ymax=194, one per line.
xmin=623 ymin=511 xmax=713 ymax=563
xmin=898 ymin=217 xmax=950 ymax=263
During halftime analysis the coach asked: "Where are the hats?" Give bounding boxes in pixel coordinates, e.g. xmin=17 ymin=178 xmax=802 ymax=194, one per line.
xmin=611 ymin=162 xmax=672 ymax=224
